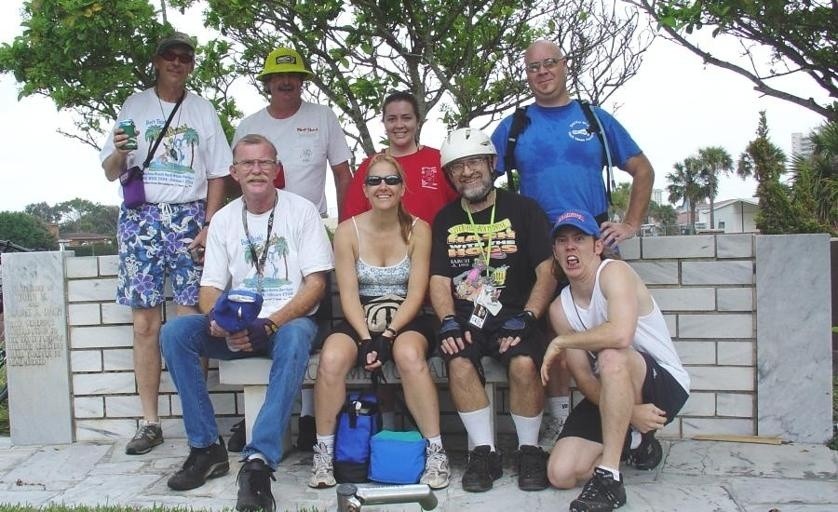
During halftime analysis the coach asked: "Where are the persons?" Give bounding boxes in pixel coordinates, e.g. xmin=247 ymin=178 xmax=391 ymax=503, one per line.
xmin=159 ymin=134 xmax=337 ymax=511
xmin=490 ymin=40 xmax=655 ymax=448
xmin=542 ymin=209 xmax=691 ymax=512
xmin=100 ymin=32 xmax=234 ymax=455
xmin=341 ymin=93 xmax=459 ymax=432
xmin=427 ymin=128 xmax=563 ymax=492
xmin=308 ymin=154 xmax=452 ymax=491
xmin=226 ymin=47 xmax=355 ymax=463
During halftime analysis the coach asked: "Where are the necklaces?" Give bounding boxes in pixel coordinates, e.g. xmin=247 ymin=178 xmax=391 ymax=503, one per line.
xmin=156 ymin=88 xmax=182 ymax=160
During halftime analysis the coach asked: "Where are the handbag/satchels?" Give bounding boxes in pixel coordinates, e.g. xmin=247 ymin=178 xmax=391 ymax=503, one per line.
xmin=361 ymin=294 xmax=405 ymax=335
xmin=367 ymin=427 xmax=429 ymax=486
xmin=330 ymin=387 xmax=382 ymax=484
xmin=117 ymin=165 xmax=147 ymax=210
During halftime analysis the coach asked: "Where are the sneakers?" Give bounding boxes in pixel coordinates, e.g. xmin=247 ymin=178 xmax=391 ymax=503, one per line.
xmin=231 ymin=457 xmax=277 ymax=512
xmin=516 ymin=444 xmax=549 ymax=491
xmin=125 ymin=420 xmax=165 ymax=456
xmin=630 ymin=427 xmax=663 ymax=471
xmin=309 ymin=442 xmax=337 ymax=489
xmin=297 ymin=413 xmax=317 ymax=451
xmin=460 ymin=443 xmax=505 ymax=494
xmin=418 ymin=443 xmax=452 ymax=489
xmin=228 ymin=417 xmax=246 ymax=452
xmin=166 ymin=433 xmax=230 ymax=492
xmin=569 ymin=465 xmax=628 ymax=511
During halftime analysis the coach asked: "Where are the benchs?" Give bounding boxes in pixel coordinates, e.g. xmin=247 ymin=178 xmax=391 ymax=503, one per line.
xmin=218 ymin=348 xmax=561 ymax=461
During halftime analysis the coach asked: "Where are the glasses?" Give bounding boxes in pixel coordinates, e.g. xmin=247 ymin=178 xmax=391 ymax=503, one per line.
xmin=156 ymin=49 xmax=194 ymax=65
xmin=526 ymin=58 xmax=555 ymax=73
xmin=365 ymin=174 xmax=402 ymax=185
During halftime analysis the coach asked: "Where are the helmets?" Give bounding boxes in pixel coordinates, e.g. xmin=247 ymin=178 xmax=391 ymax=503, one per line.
xmin=437 ymin=127 xmax=498 ymax=169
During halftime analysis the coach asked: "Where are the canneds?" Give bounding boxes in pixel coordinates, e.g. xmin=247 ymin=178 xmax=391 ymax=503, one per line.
xmin=119 ymin=119 xmax=138 ymax=150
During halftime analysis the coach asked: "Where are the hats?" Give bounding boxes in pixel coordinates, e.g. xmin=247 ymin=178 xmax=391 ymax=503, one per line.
xmin=254 ymin=47 xmax=315 ymax=83
xmin=213 ymin=287 xmax=264 ymax=335
xmin=550 ymin=207 xmax=601 ymax=237
xmin=154 ymin=32 xmax=198 ymax=53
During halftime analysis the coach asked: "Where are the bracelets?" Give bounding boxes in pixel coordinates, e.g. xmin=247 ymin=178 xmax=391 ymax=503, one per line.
xmin=202 ymin=221 xmax=209 ymax=228
xmin=386 ymin=327 xmax=396 ymax=337
